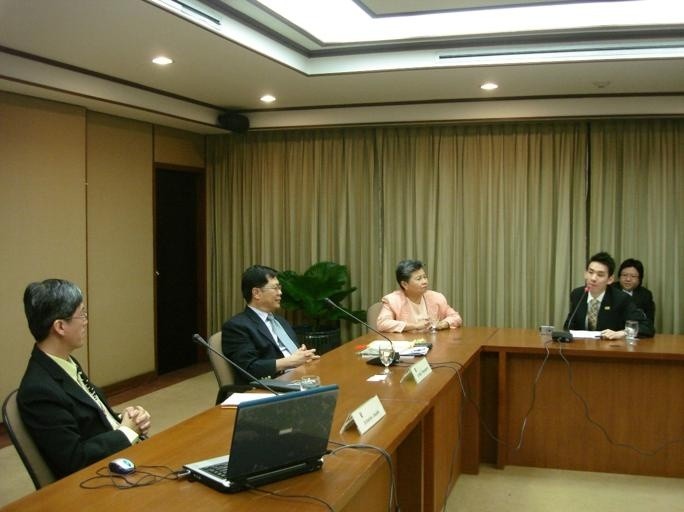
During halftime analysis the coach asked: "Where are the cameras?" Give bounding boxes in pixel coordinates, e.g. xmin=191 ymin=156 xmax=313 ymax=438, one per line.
xmin=541 ymin=326 xmax=553 ymax=335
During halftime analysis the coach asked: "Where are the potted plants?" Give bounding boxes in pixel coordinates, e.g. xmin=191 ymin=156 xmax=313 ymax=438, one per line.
xmin=276 ymin=261 xmax=367 ymax=355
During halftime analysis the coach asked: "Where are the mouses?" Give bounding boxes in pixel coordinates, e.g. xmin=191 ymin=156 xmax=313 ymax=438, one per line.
xmin=109 ymin=458 xmax=135 ymax=474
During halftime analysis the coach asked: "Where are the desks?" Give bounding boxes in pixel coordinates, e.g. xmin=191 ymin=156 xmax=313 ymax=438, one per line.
xmin=250 ymin=326 xmax=499 ymax=512
xmin=482 ymin=327 xmax=684 ymax=478
xmin=0 ymin=371 xmax=432 ymax=511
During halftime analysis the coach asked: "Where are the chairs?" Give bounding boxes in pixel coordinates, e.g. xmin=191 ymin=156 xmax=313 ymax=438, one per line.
xmin=2 ymin=389 xmax=55 ymax=492
xmin=207 ymin=331 xmax=234 ymax=405
xmin=366 ymin=301 xmax=383 ymax=333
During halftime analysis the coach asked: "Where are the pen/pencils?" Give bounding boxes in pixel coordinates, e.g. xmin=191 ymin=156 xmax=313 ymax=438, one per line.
xmin=595 ymin=335 xmax=601 ymax=338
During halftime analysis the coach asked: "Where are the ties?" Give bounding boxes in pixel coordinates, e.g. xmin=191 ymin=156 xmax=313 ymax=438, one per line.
xmin=268 ymin=314 xmax=298 ymax=355
xmin=78 ymin=367 xmax=104 ymax=410
xmin=588 ymin=300 xmax=597 ymax=330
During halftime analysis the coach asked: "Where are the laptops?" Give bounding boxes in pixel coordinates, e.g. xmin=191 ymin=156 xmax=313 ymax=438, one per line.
xmin=182 ymin=382 xmax=339 ymax=494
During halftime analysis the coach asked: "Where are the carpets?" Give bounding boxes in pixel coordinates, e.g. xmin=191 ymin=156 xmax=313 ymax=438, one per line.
xmin=147 ymin=374 xmax=684 ymax=512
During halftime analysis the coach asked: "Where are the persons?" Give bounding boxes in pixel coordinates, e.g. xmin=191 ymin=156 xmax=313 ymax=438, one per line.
xmin=16 ymin=278 xmax=152 ymax=478
xmin=221 ymin=265 xmax=321 ymax=382
xmin=612 ymin=259 xmax=656 ymax=324
xmin=377 ymin=259 xmax=462 ymax=332
xmin=564 ymin=252 xmax=655 ymax=339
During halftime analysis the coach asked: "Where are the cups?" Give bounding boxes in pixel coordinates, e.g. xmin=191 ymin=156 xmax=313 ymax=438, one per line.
xmin=624 ymin=320 xmax=638 ymax=340
xmin=300 ymin=375 xmax=320 ymax=389
xmin=378 ymin=341 xmax=394 ymax=376
xmin=429 ymin=313 xmax=440 ymax=335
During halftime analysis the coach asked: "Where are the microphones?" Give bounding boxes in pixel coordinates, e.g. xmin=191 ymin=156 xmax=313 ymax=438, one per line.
xmin=324 ymin=296 xmax=400 ymax=368
xmin=552 ymin=283 xmax=592 ymax=343
xmin=193 ymin=334 xmax=278 ymax=396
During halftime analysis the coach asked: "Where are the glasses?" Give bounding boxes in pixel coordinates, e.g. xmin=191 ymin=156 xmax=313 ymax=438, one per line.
xmin=59 ymin=312 xmax=88 ymax=320
xmin=256 ymin=284 xmax=282 ymax=290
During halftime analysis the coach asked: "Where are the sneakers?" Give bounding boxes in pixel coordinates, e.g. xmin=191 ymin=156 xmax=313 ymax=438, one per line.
xmin=620 ymin=272 xmax=639 ymax=279
